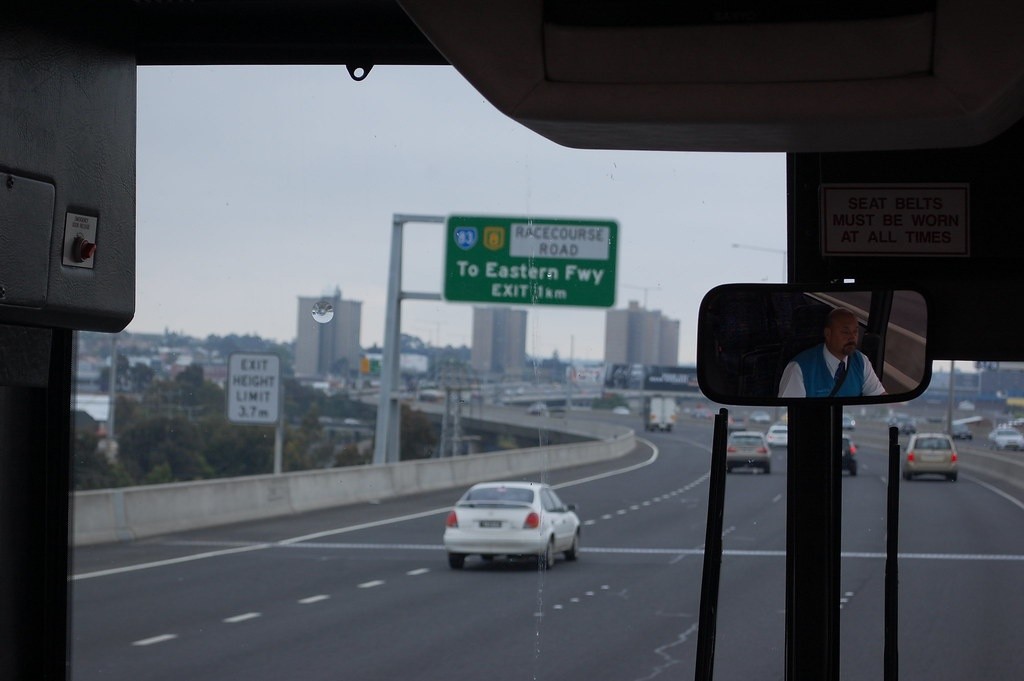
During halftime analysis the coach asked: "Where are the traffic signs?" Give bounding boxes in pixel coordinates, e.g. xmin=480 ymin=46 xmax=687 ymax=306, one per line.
xmin=442 ymin=212 xmax=616 ymax=307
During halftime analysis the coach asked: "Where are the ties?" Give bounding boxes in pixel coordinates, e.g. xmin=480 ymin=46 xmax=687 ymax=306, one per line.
xmin=834 ymin=362 xmax=845 ymax=384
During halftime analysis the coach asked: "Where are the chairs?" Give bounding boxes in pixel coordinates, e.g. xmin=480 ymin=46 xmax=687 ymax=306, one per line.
xmin=709 ymin=288 xmax=834 ymax=397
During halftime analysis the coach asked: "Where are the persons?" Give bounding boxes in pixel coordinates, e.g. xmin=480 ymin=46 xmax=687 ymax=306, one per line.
xmin=774 ymin=307 xmax=888 ymax=400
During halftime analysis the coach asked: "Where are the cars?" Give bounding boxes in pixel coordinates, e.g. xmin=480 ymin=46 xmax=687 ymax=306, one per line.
xmin=843 ymin=410 xmax=1023 ymax=481
xmin=439 ymin=480 xmax=583 ymax=572
xmin=415 ymin=389 xmax=788 ymax=473
